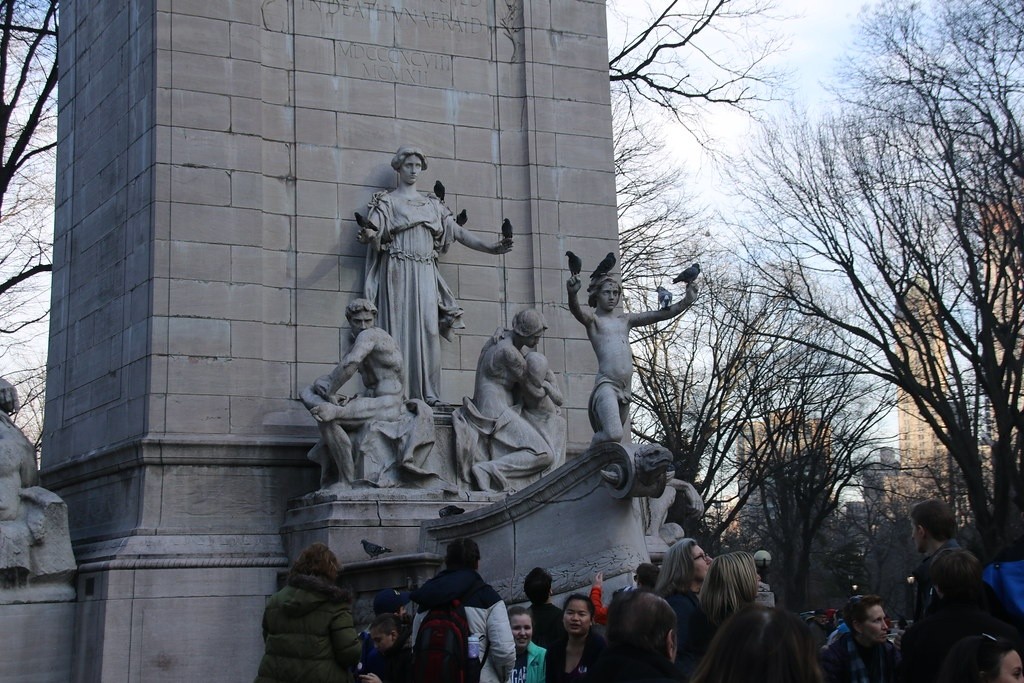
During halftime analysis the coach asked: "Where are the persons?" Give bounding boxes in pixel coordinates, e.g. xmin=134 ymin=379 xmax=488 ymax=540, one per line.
xmin=472 ymin=307 xmax=566 ymax=493
xmin=357 ymin=147 xmax=514 ymax=408
xmin=361 ymin=501 xmax=1024 ymax=683
xmin=567 ymin=272 xmax=700 ymax=449
xmin=254 ymin=543 xmax=358 ymax=683
xmin=299 ymin=299 xmax=406 ymax=490
xmin=0 ymin=379 xmax=37 ymax=588
xmin=640 ymin=471 xmax=704 ymax=536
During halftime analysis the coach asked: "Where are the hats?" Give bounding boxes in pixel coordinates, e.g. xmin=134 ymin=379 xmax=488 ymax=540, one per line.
xmin=374 ymin=589 xmax=412 ymax=614
xmin=815 ymin=609 xmax=827 ymax=615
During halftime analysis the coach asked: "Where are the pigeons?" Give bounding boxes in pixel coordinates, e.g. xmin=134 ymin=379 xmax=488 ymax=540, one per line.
xmin=564 ymin=250 xmax=582 ymax=277
xmin=590 ymin=252 xmax=617 ymax=281
xmin=456 ymin=209 xmax=469 ymax=227
xmin=433 ymin=180 xmax=445 ymax=203
xmin=359 ymin=538 xmax=394 ymax=560
xmin=501 ymin=217 xmax=513 ymax=238
xmin=655 ymin=285 xmax=673 ymax=312
xmin=354 ymin=212 xmax=379 ymax=232
xmin=439 ymin=504 xmax=466 ymax=518
xmin=672 ymin=263 xmax=701 ymax=285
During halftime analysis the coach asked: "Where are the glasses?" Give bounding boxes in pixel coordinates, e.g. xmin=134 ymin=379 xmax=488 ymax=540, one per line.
xmin=978 ymin=633 xmax=996 ymax=657
xmin=850 ymin=597 xmax=860 ymax=604
xmin=634 ymin=575 xmax=637 ymax=582
xmin=694 ymin=552 xmax=708 ymax=561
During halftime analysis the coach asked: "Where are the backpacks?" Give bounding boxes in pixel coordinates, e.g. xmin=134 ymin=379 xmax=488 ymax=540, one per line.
xmin=412 ymin=579 xmax=491 ymax=683
xmin=351 ymin=632 xmax=377 ymax=673
